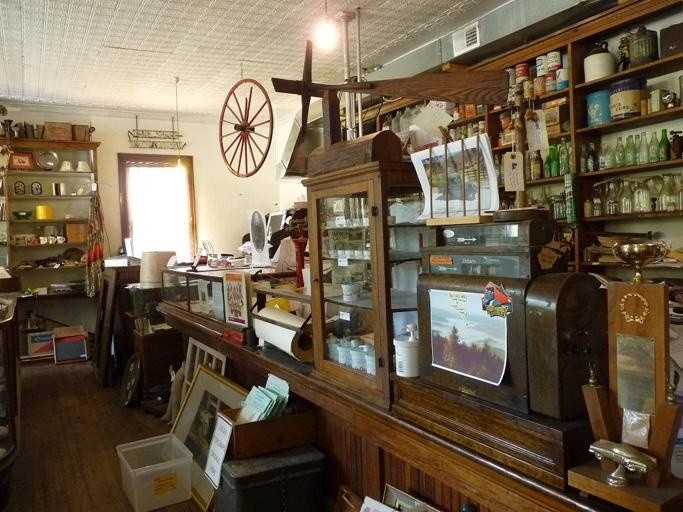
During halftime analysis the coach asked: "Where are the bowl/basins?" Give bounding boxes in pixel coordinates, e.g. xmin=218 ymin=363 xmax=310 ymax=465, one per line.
xmin=597 ymin=235 xmax=630 ymax=247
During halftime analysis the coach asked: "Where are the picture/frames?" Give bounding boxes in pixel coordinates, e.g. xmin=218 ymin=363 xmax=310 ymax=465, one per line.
xmin=380 ymin=483 xmax=445 ymax=512
xmin=8 ymin=152 xmax=33 ymax=170
xmin=160 ymin=362 xmax=250 ymax=512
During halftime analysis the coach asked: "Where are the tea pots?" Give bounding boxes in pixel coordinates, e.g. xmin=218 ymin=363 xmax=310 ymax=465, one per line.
xmin=583 ymin=41 xmax=625 ymax=83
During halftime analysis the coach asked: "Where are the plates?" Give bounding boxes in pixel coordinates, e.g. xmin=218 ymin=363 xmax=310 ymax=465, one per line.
xmin=36 ymin=150 xmax=59 ymax=171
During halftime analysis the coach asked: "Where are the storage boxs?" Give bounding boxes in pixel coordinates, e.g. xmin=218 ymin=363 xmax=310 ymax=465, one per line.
xmin=212 ymin=446 xmax=327 ymax=512
xmin=40 ymin=122 xmax=71 ymax=141
xmin=114 ymin=431 xmax=193 ymax=512
xmin=62 ymin=223 xmax=89 ymax=244
xmin=71 ymin=125 xmax=90 ymax=141
xmin=26 ymin=330 xmax=53 ymax=356
xmin=218 ymin=396 xmax=318 ymax=458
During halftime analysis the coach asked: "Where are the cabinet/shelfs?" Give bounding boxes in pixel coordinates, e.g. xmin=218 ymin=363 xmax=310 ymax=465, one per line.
xmin=0 ymin=139 xmax=102 ymax=303
xmin=486 ymin=21 xmax=575 ymax=270
xmin=339 ymin=68 xmax=484 ymax=157
xmin=301 ymin=160 xmax=434 ymax=412
xmin=569 ymin=0 xmax=682 ymax=274
xmin=0 ymin=288 xmax=22 ymax=478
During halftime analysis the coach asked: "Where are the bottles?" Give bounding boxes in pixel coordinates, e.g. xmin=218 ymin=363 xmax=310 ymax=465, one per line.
xmin=381 ymin=105 xmax=421 ymax=134
xmin=580 ymin=125 xmax=682 ymax=174
xmin=583 ymin=173 xmax=683 ymax=218
xmin=525 ymin=183 xmax=565 ymax=218
xmin=529 ymin=136 xmax=572 ymax=179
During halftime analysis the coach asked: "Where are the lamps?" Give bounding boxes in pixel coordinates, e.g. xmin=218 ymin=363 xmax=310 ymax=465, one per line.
xmin=308 ymin=0 xmax=340 ymax=55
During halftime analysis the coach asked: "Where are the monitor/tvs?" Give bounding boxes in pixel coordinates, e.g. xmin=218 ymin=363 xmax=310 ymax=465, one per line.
xmin=266 ymin=213 xmax=286 ymax=236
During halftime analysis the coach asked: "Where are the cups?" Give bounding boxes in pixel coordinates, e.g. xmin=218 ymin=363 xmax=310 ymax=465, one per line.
xmin=38 ymin=236 xmax=65 ymax=245
